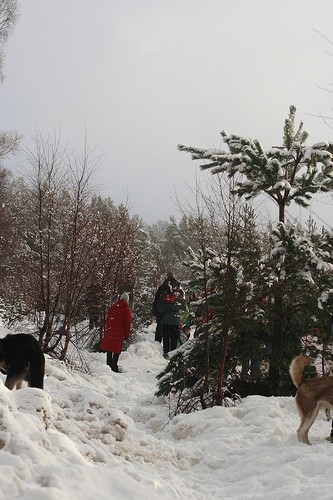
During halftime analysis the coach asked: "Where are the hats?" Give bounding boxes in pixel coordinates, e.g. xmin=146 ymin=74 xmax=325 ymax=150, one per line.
xmin=120 ymin=291 xmax=129 ymax=302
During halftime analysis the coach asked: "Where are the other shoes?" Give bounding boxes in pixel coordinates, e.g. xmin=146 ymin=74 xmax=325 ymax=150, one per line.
xmin=164 ymin=353 xmax=168 ymax=359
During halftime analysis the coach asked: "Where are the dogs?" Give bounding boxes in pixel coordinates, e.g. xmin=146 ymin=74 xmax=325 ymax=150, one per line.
xmin=0 ymin=330 xmax=48 ymax=396
xmin=289 ymin=352 xmax=333 ymax=445
